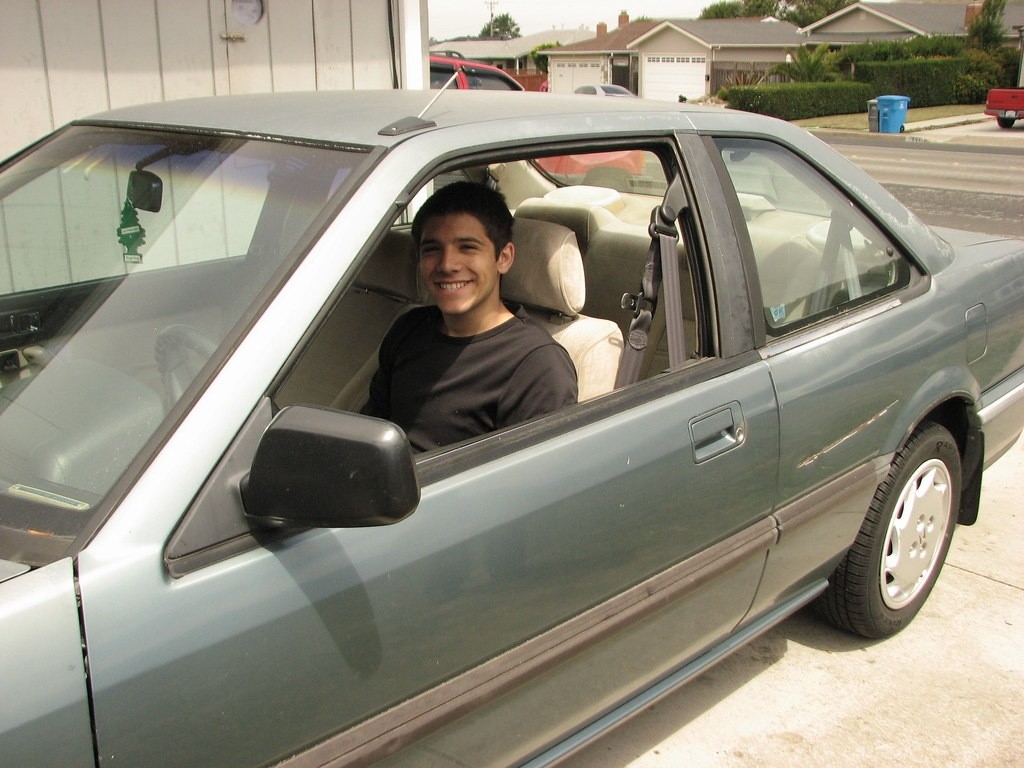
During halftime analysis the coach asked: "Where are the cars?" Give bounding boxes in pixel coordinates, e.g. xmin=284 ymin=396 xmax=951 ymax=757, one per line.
xmin=571 ymin=83 xmax=636 ymax=96
xmin=0 ymin=88 xmax=1023 ymax=768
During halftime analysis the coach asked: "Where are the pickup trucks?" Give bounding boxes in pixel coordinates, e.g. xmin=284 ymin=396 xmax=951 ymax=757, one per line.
xmin=984 ymin=86 xmax=1024 ymax=129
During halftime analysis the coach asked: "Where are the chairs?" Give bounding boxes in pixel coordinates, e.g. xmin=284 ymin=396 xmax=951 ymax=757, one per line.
xmin=262 ymin=213 xmax=625 ymax=475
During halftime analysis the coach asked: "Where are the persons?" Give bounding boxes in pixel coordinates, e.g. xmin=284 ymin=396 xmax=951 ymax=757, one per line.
xmin=360 ymin=180 xmax=577 ymax=456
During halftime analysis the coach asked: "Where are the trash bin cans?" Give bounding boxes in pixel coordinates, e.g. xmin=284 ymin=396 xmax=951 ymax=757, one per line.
xmin=866 ymin=98 xmax=879 ymax=133
xmin=875 ymin=95 xmax=911 ymax=133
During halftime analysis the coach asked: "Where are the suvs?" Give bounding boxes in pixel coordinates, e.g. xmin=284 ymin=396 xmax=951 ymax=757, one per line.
xmin=430 ymin=49 xmax=530 ymax=91
